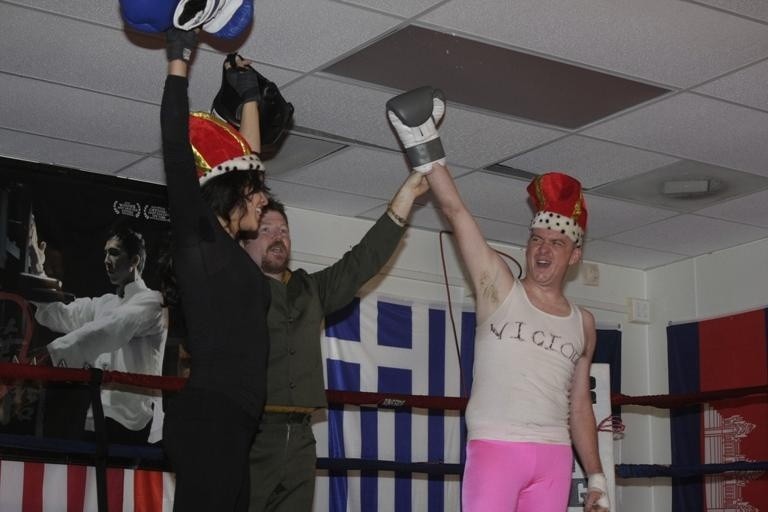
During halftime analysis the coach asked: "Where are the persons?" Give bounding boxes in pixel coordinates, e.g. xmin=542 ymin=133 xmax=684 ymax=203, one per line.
xmin=157 ymin=27 xmax=274 ymax=511
xmin=384 ymin=84 xmax=611 ymax=511
xmin=25 ymin=212 xmax=171 ymax=446
xmin=241 ymin=170 xmax=433 ymax=512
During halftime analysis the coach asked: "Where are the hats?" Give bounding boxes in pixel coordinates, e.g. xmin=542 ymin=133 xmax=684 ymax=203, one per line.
xmin=190 ymin=107 xmax=264 ymax=185
xmin=526 ymin=172 xmax=588 ymax=248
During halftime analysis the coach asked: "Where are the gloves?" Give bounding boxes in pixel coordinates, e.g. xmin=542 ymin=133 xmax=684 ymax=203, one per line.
xmin=121 ymin=0 xmax=253 ymax=61
xmin=386 ymin=85 xmax=446 ymax=174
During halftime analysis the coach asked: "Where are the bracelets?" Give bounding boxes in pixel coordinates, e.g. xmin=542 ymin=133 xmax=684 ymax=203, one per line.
xmin=386 ymin=204 xmax=409 ymax=226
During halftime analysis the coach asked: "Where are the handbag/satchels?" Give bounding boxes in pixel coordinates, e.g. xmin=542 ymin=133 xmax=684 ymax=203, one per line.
xmin=210 ymin=53 xmax=294 ymax=160
